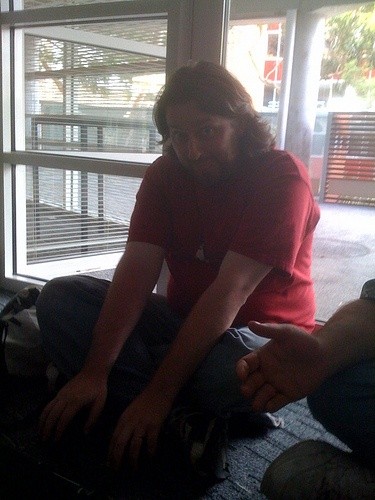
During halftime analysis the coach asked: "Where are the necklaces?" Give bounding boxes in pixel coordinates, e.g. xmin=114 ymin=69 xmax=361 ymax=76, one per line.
xmin=191 ymin=154 xmax=242 ymax=262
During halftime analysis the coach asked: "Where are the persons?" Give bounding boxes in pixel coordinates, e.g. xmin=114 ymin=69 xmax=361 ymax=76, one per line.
xmin=32 ymin=58 xmax=322 ymax=490
xmin=235 ymin=270 xmax=375 ymax=497
